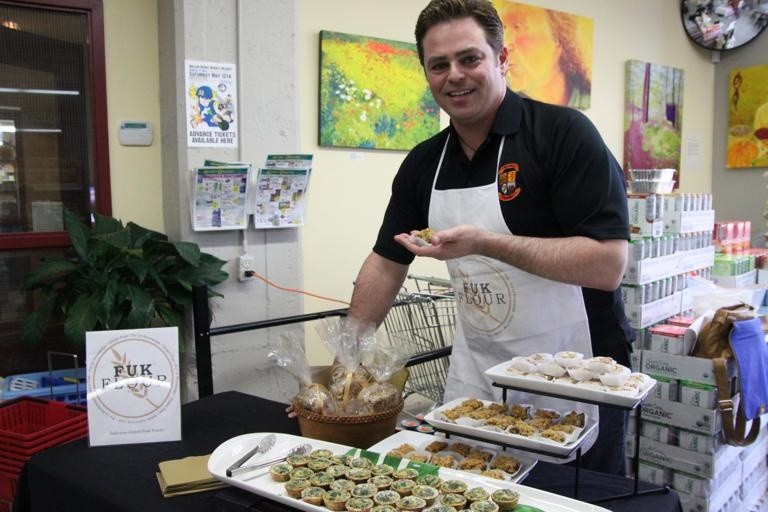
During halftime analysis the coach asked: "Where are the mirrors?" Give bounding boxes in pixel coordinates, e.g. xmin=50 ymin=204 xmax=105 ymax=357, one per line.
xmin=679 ymin=0 xmax=767 ymax=51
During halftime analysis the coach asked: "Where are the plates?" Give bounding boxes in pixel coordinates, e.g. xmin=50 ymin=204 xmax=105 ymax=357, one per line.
xmin=364 ymin=428 xmax=540 ymax=489
xmin=422 ymin=396 xmax=599 ymax=459
xmin=207 ymin=432 xmax=606 ymax=509
xmin=483 ymin=356 xmax=659 ymax=409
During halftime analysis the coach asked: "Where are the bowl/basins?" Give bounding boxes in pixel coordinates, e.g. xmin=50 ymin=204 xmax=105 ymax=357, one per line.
xmin=628 ymin=168 xmax=677 ymax=180
xmin=628 ymin=179 xmax=677 ymax=195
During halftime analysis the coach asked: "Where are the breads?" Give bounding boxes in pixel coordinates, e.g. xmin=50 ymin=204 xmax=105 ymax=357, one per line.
xmin=329 ymin=364 xmax=374 ymax=399
xmin=301 ymin=386 xmax=341 ymax=417
xmin=357 ymin=384 xmax=399 ymax=412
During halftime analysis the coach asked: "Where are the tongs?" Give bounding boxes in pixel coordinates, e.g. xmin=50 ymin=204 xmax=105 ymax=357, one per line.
xmin=224 ymin=433 xmax=314 ymax=481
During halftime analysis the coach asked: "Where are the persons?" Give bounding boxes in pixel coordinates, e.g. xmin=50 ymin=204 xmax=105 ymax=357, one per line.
xmin=706 ymin=0 xmax=715 ymax=14
xmin=729 ymin=0 xmax=740 ymax=19
xmin=285 ymin=1 xmax=634 ymax=477
xmin=695 ymin=3 xmax=707 ymax=16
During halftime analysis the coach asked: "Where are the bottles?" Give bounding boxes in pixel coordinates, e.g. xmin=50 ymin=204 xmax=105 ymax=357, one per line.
xmin=621 ymin=268 xmax=717 ymax=329
xmin=631 ymin=191 xmax=713 ymax=237
xmin=623 ymin=236 xmax=716 ymax=285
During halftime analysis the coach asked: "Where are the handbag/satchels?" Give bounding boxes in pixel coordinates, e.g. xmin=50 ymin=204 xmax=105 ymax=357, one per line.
xmin=692 ymin=303 xmax=768 ymax=446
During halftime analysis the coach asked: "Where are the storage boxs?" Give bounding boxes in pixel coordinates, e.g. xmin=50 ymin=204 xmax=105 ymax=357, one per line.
xmin=678 ymin=437 xmax=768 ymax=512
xmin=715 ymin=269 xmax=758 ymax=290
xmin=640 ymin=397 xmax=724 ymax=437
xmin=663 ymin=209 xmax=714 ymax=235
xmin=628 ymin=197 xmax=665 ymax=238
xmin=640 ymin=349 xmax=718 ymax=386
xmin=1 ymin=396 xmax=89 ymax=511
xmin=724 ymin=473 xmax=768 ymax=511
xmin=619 ymin=245 xmax=715 ymax=287
xmin=624 ymin=288 xmax=693 ymax=331
xmin=638 ymin=438 xmax=742 ymax=482
